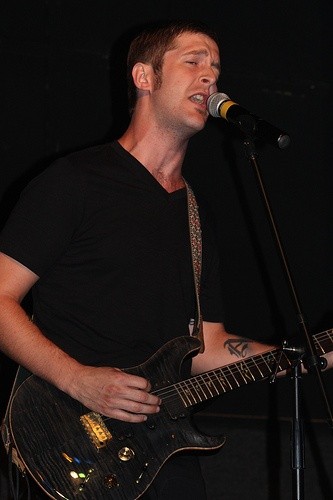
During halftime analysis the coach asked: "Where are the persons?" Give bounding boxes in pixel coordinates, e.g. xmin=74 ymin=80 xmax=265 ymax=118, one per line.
xmin=1 ymin=17 xmax=333 ymax=500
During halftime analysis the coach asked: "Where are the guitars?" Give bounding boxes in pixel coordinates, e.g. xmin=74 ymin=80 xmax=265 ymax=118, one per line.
xmin=0 ymin=327 xmax=332 ymax=500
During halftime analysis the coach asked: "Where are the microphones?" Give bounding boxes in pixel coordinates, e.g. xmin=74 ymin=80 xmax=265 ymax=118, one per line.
xmin=206 ymin=92 xmax=292 ymax=149
